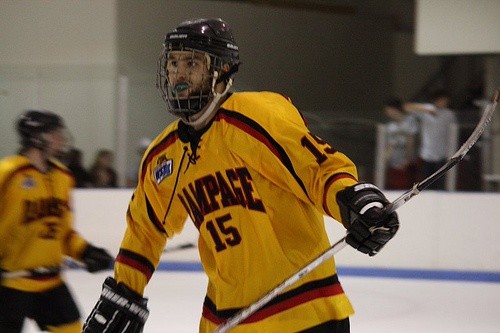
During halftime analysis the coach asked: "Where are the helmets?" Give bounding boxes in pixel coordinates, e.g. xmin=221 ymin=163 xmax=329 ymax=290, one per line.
xmin=16 ymin=111 xmax=64 ymax=148
xmin=163 ymin=17 xmax=240 ymax=68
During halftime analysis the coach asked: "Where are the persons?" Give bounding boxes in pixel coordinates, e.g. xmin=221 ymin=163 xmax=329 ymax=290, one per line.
xmin=126 ymin=138 xmax=154 ymax=187
xmin=402 ymin=88 xmax=458 ymax=190
xmin=78 ymin=17 xmax=399 ymax=333
xmin=381 ymin=99 xmax=417 ymax=188
xmin=89 ymin=148 xmax=118 ymax=189
xmin=53 ymin=137 xmax=84 ymax=189
xmin=1 ymin=111 xmax=116 ymax=333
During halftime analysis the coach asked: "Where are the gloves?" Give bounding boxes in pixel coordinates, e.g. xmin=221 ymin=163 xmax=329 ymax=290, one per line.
xmin=335 ymin=182 xmax=400 ymax=257
xmin=78 ymin=243 xmax=115 ymax=273
xmin=80 ymin=276 xmax=149 ymax=333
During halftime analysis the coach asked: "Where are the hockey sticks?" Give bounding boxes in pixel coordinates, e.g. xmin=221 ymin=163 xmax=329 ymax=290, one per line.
xmin=0 ymin=242 xmax=198 ymax=282
xmin=213 ymin=91 xmax=500 ymax=333
